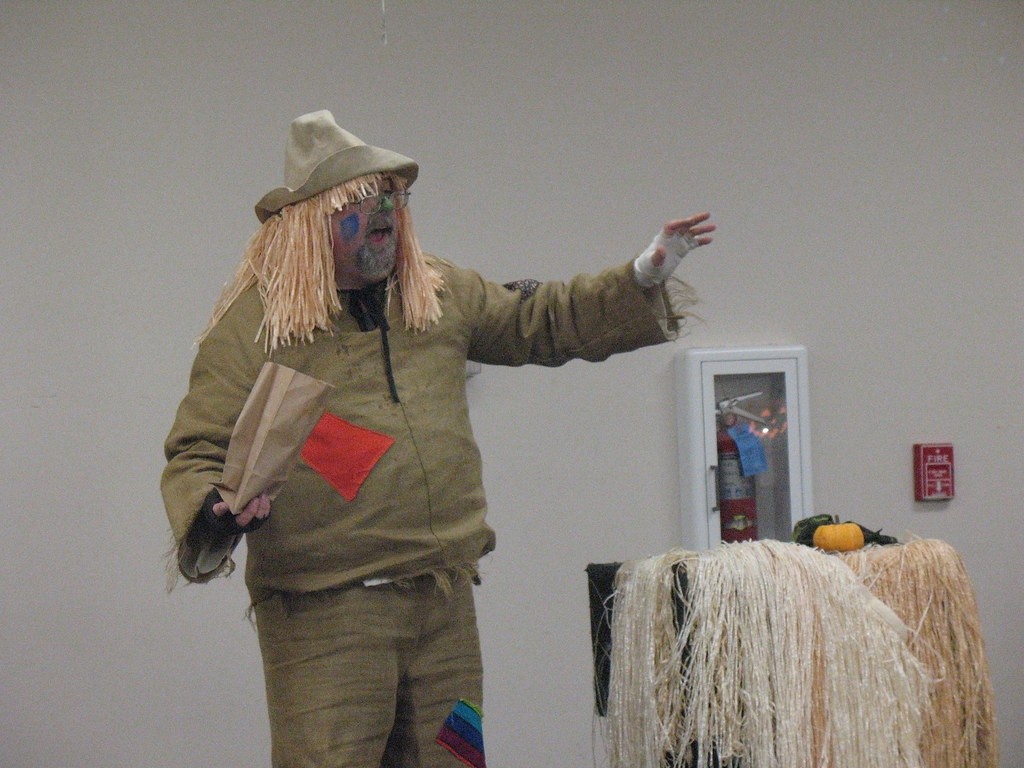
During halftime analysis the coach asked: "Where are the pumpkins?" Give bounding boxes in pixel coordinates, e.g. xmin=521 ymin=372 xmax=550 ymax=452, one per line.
xmin=813 ymin=514 xmax=864 ymax=554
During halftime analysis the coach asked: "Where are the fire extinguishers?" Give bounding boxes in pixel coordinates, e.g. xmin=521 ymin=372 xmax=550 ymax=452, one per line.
xmin=710 ymin=390 xmax=769 ymax=543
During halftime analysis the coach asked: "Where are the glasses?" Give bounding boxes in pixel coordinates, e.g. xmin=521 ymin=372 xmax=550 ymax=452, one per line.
xmin=344 ymin=191 xmax=412 ymax=215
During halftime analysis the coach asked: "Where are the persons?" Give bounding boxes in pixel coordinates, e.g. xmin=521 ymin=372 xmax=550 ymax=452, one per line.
xmin=159 ymin=110 xmax=716 ymax=768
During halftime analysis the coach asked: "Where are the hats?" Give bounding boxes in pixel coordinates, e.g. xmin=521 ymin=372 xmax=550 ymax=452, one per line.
xmin=255 ymin=110 xmax=420 ymax=224
